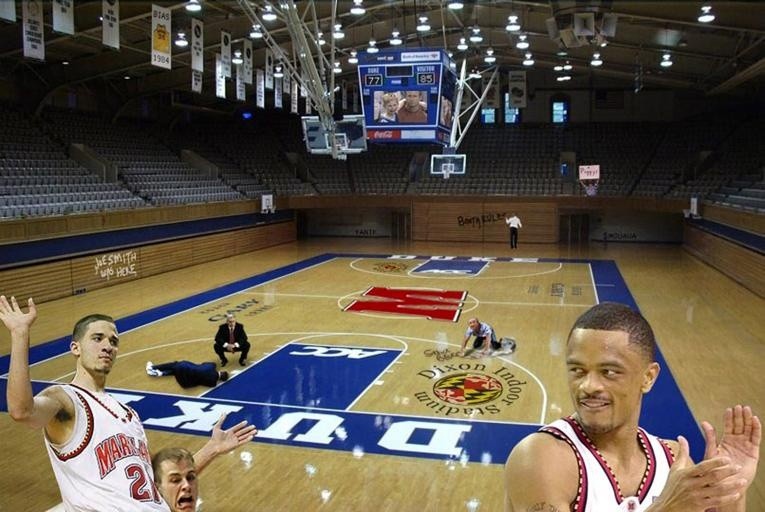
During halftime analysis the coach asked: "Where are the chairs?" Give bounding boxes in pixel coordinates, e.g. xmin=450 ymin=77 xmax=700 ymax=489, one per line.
xmin=221 ymin=110 xmax=578 ymax=196
xmin=0 ymin=100 xmax=250 ymax=223
xmin=579 ymin=114 xmax=765 ymax=216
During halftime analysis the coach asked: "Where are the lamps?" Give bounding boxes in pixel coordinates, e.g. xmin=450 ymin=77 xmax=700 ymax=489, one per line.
xmin=167 ymin=1 xmax=717 ymax=104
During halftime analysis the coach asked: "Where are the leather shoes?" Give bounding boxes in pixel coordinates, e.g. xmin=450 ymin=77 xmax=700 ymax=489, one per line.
xmin=220 ymin=360 xmax=247 ymax=368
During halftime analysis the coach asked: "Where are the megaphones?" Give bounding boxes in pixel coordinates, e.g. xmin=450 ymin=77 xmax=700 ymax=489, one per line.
xmin=545 ymin=12 xmax=617 ymax=48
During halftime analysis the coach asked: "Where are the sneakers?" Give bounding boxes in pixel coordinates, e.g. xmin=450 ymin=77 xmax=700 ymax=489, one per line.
xmin=144 ymin=359 xmax=160 ymax=377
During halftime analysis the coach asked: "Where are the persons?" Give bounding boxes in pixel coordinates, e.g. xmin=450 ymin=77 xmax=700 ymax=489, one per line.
xmin=212 ymin=311 xmax=252 ymax=368
xmin=143 ymin=356 xmax=230 ymax=391
xmin=501 ymin=300 xmax=764 ymax=510
xmin=373 ymin=93 xmax=401 ymax=126
xmin=578 ymin=177 xmax=602 ymax=198
xmin=397 ymin=91 xmax=428 ymax=123
xmin=503 ymin=211 xmax=524 ymax=247
xmin=0 ymin=292 xmax=261 ymax=511
xmin=453 ymin=316 xmax=506 ymax=358
xmin=151 ymin=446 xmax=205 ymax=512
